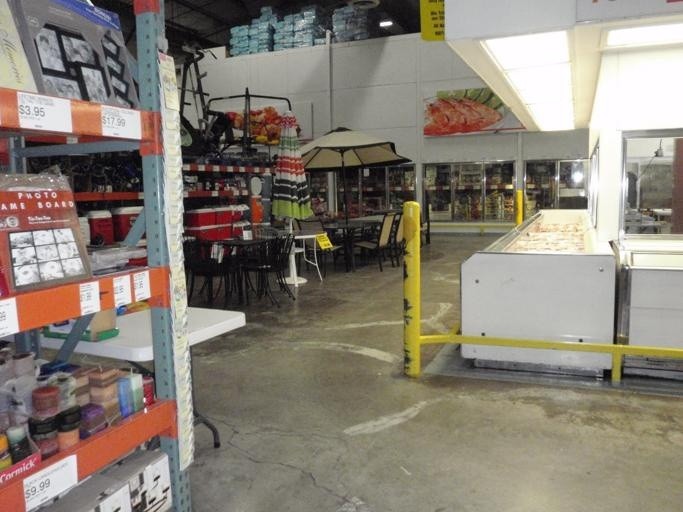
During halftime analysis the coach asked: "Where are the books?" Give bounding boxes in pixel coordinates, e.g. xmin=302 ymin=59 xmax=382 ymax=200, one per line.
xmin=85 ymin=204 xmax=249 ymax=264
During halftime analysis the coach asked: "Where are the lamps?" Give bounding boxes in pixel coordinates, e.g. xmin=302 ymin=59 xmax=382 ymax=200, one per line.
xmin=183 ymin=211 xmax=401 ymax=307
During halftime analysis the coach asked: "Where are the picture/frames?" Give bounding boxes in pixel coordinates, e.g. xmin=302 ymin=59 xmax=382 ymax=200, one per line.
xmin=41 ymin=306 xmax=247 ymax=449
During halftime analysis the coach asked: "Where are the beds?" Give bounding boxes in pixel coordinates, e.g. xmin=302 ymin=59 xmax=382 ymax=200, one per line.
xmin=300 ymin=125 xmax=413 ymax=224
xmin=270 ymin=109 xmax=314 ymax=276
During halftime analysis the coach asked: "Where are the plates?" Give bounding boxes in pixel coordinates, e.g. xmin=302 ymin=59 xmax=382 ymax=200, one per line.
xmin=2 ymin=1 xmax=194 ymax=512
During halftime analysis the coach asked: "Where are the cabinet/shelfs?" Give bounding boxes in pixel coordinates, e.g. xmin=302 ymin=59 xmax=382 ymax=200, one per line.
xmin=0 ymin=384 xmax=80 ymax=471
